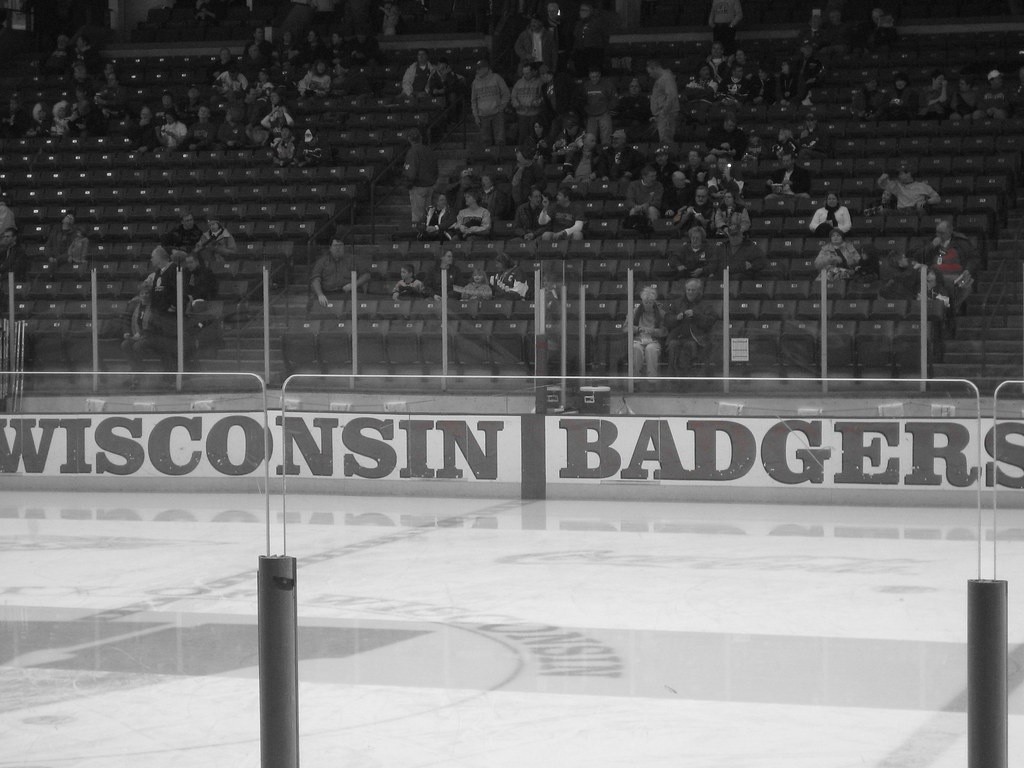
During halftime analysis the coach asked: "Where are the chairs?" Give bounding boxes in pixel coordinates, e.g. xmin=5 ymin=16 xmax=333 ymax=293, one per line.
xmin=0 ymin=0 xmax=1024 ymax=389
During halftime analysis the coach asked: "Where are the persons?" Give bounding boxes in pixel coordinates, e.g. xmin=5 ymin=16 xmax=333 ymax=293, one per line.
xmin=0 ymin=0 xmax=1024 ymax=395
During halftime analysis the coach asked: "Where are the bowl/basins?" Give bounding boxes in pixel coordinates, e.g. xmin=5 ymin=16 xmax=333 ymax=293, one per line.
xmin=772 ymin=184 xmax=783 ymax=193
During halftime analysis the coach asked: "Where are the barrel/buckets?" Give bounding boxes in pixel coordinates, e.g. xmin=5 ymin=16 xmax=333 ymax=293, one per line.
xmin=547 ymin=387 xmax=561 ymax=408
xmin=580 ymin=386 xmax=611 ymax=414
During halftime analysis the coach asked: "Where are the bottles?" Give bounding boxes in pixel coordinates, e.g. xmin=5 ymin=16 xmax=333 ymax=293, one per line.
xmin=937 ymin=253 xmax=942 ymax=265
